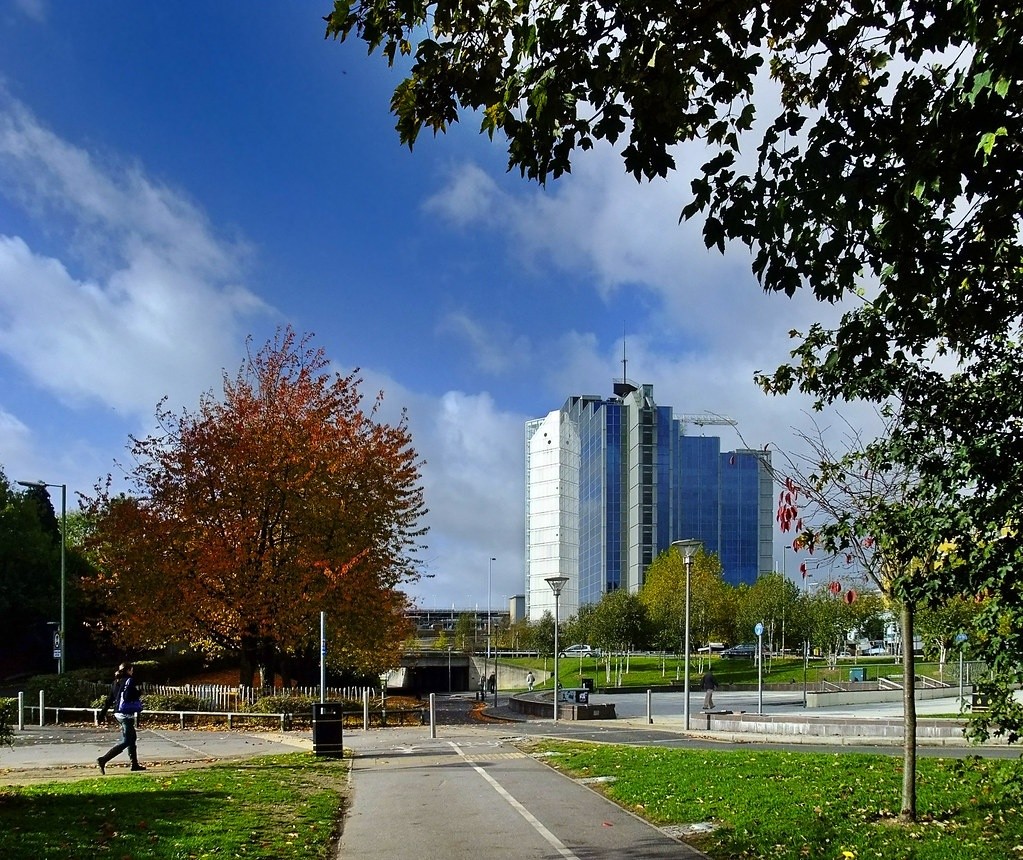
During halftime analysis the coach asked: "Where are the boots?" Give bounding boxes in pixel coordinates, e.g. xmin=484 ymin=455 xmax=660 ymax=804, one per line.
xmin=96 ymin=744 xmax=123 ymax=775
xmin=128 ymin=745 xmax=146 ymax=771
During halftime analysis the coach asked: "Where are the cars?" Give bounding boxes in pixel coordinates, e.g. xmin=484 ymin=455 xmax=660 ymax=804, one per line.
xmin=839 ymin=652 xmax=851 ymax=657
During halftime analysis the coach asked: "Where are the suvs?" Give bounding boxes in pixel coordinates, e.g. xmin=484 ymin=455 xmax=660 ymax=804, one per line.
xmin=720 ymin=644 xmax=757 ymax=657
xmin=559 ymin=644 xmax=595 ymax=658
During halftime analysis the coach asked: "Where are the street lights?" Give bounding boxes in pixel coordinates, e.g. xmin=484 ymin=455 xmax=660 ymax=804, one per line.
xmin=18 ymin=480 xmax=66 ymax=674
xmin=672 ymin=538 xmax=704 ymax=730
xmin=803 ymin=558 xmax=818 ymax=655
xmin=545 ymin=576 xmax=569 ymax=721
xmin=781 ymin=546 xmax=791 ymax=658
xmin=488 ymin=557 xmax=495 ymax=659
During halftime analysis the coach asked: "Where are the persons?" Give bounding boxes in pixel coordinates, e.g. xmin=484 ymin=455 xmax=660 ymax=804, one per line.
xmin=97 ymin=663 xmax=146 ymax=775
xmin=700 ymin=668 xmax=717 ymax=709
xmin=527 ymin=672 xmax=535 ymax=691
xmin=489 ymin=671 xmax=495 ymax=694
xmin=479 ymin=674 xmax=485 ymax=691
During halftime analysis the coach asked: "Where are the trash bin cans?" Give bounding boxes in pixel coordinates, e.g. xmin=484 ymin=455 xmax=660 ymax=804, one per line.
xmin=582 ymin=678 xmax=593 ymax=693
xmin=312 ymin=703 xmax=342 ymax=758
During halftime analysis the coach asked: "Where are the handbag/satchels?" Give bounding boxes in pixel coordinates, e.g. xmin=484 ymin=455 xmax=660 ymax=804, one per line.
xmin=119 ymin=678 xmax=143 ymax=713
